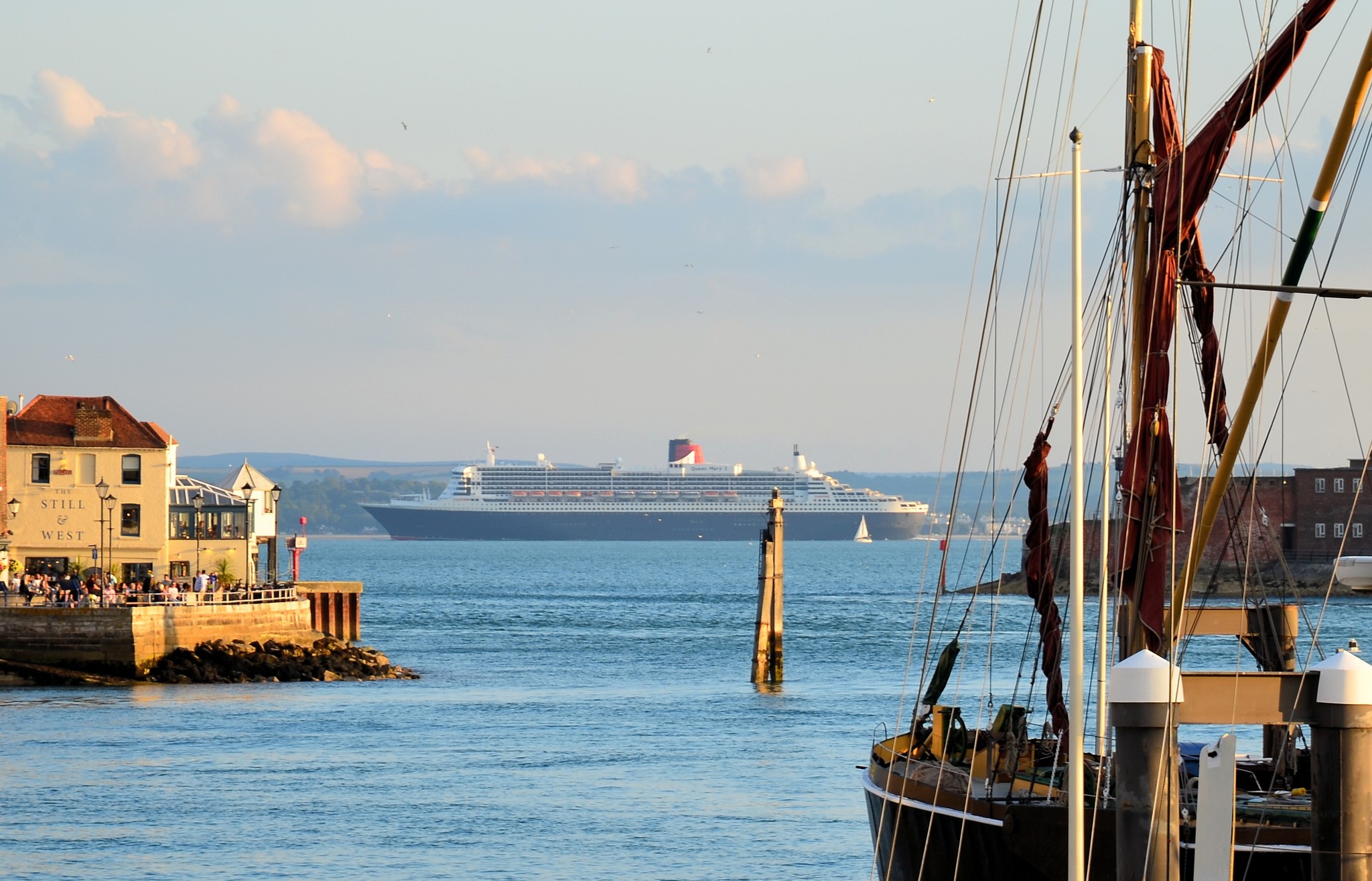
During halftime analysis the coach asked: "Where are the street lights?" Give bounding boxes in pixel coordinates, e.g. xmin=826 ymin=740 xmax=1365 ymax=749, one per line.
xmin=271 ymin=483 xmax=282 ymax=582
xmin=104 ymin=494 xmax=117 ymax=584
xmin=96 ymin=476 xmax=109 ymax=607
xmin=241 ymin=483 xmax=255 ymax=592
xmin=191 ymin=491 xmax=204 ymax=570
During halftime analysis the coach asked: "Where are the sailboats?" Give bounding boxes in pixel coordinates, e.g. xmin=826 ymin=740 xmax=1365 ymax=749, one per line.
xmin=853 ymin=514 xmax=873 ymax=544
xmin=863 ymin=0 xmax=1372 ymax=881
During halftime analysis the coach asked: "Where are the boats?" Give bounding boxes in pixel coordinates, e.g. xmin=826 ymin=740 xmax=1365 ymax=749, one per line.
xmin=704 ymin=491 xmax=719 ymax=498
xmin=617 ymin=492 xmax=635 ymax=500
xmin=564 ymin=492 xmax=581 ymax=497
xmin=529 ymin=491 xmax=545 ymax=497
xmin=681 ymin=492 xmax=700 ymax=499
xmin=660 ymin=491 xmax=679 ymax=499
xmin=598 ymin=492 xmax=615 ymax=498
xmin=546 ymin=491 xmax=562 ymax=497
xmin=511 ymin=491 xmax=527 ymax=497
xmin=1333 ymin=555 xmax=1372 ymax=592
xmin=722 ymin=490 xmax=737 ymax=498
xmin=637 ymin=492 xmax=658 ymax=500
xmin=582 ymin=492 xmax=594 ymax=498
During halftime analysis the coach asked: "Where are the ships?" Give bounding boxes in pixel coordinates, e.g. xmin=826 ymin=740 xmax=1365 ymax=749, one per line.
xmin=354 ymin=437 xmax=930 ymax=544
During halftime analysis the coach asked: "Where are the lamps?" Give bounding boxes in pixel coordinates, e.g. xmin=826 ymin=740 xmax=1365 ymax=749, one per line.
xmin=4 ymin=496 xmax=22 ymax=521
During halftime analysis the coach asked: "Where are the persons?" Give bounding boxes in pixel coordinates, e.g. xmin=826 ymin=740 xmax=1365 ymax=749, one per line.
xmin=179 ymin=521 xmax=239 ymax=539
xmin=12 ymin=568 xmax=256 ymax=607
